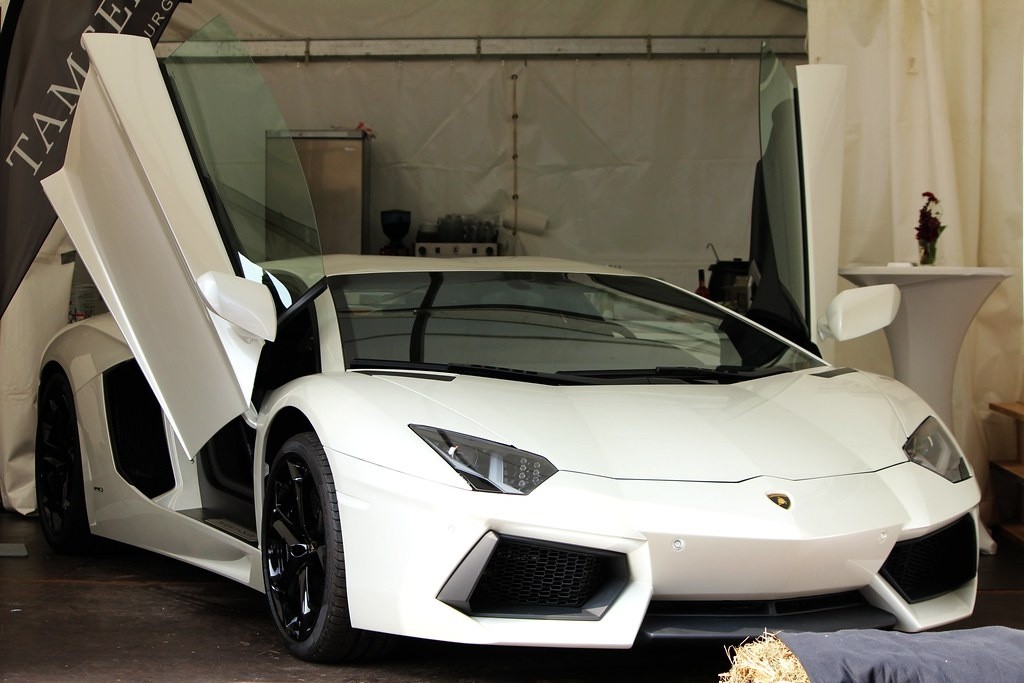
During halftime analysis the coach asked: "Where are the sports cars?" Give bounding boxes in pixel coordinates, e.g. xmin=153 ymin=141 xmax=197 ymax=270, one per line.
xmin=0 ymin=32 xmax=996 ymax=670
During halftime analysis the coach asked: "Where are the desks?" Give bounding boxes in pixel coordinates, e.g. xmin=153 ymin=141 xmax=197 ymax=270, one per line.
xmin=839 ymin=266 xmax=1014 ymax=554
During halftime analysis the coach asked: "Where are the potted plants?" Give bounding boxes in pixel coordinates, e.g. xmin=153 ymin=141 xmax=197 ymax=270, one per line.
xmin=415 ymin=213 xmax=501 ymax=243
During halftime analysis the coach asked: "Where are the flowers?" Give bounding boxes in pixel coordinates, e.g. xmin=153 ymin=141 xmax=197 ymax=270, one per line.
xmin=915 ymin=191 xmax=946 ymax=241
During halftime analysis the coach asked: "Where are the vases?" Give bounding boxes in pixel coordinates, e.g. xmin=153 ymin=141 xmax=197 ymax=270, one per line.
xmin=917 ymin=239 xmax=937 ymax=265
